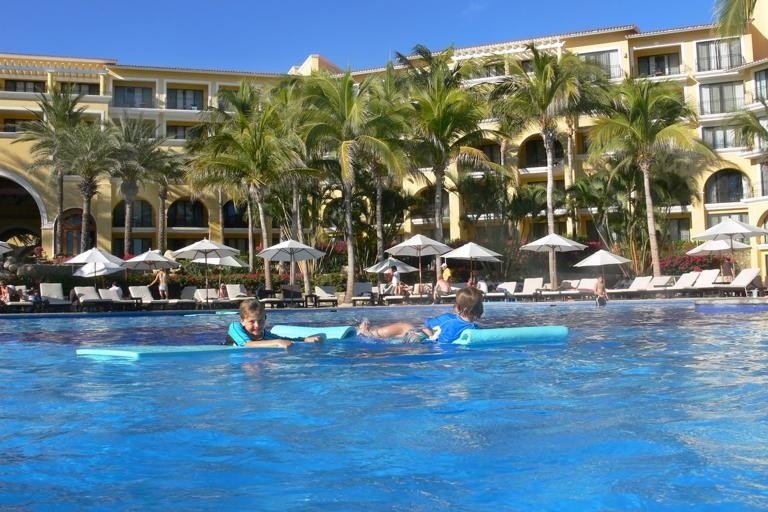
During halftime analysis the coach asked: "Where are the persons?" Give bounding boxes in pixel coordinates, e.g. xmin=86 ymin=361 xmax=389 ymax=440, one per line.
xmin=594 ymin=275 xmax=609 ymax=309
xmin=0 ymin=280 xmax=42 ymax=306
xmin=432 ymin=262 xmax=488 ymax=305
xmin=358 ymin=288 xmax=484 ymax=345
xmin=384 ymin=265 xmax=412 ymax=297
xmin=721 ymin=256 xmax=735 ymax=296
xmin=218 ymin=299 xmax=322 ymax=349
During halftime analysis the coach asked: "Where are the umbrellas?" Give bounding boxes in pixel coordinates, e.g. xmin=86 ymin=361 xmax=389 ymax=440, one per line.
xmin=256 ymin=238 xmax=326 ymax=306
xmin=64 ymin=236 xmax=250 ymax=309
xmin=685 ymin=237 xmax=752 ymax=281
xmin=519 ymin=232 xmax=589 ymax=288
xmin=0 ymin=241 xmax=14 ymax=256
xmin=692 ymin=218 xmax=768 ymax=281
xmin=363 ymin=233 xmax=504 ymax=284
xmin=573 ymin=248 xmax=633 ymax=287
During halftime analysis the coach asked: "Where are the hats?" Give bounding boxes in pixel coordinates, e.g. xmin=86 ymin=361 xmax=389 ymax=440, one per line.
xmin=439 ymin=263 xmax=448 ymax=268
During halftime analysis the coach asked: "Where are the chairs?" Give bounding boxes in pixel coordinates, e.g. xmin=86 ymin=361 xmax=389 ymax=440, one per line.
xmin=352 ymin=279 xmax=598 ymax=306
xmin=1 ymin=282 xmax=338 ymax=315
xmin=605 ymin=267 xmax=760 ymax=300
